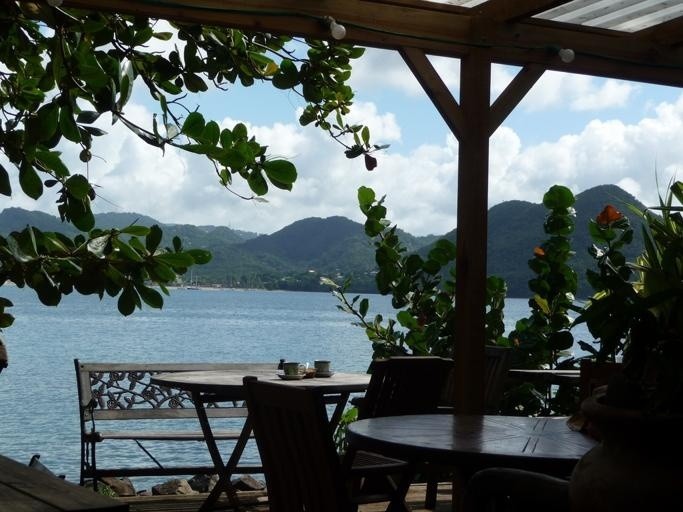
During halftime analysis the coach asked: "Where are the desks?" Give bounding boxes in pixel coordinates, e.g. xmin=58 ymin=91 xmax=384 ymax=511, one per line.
xmin=151 ymin=369 xmax=388 ymax=512
xmin=345 ymin=415 xmax=600 ymax=512
xmin=0 ymin=455 xmax=129 ymax=512
xmin=507 ymin=368 xmax=582 ymax=383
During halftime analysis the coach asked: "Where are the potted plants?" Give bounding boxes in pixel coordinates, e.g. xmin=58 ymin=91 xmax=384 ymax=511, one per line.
xmin=582 ymin=251 xmax=683 ymax=464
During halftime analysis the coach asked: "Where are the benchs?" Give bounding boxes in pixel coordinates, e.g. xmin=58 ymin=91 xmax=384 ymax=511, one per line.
xmin=75 ymin=361 xmax=308 ymax=492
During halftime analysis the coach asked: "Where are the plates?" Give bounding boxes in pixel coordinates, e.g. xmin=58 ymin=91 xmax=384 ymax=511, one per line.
xmin=316 ymin=370 xmax=336 ymax=377
xmin=276 ymin=373 xmax=306 ymax=379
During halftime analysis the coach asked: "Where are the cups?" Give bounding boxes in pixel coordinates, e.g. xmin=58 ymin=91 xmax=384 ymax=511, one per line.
xmin=284 ymin=362 xmax=306 ymax=375
xmin=313 ymin=360 xmax=330 ymax=371
